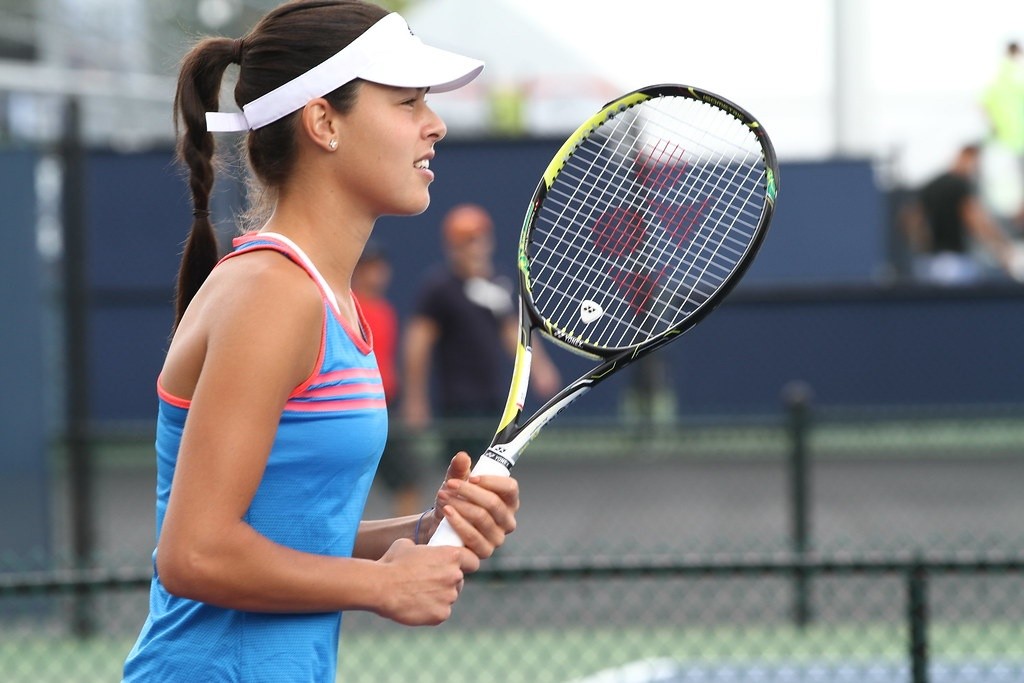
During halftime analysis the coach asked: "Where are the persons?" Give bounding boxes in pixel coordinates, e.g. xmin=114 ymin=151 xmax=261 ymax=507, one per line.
xmin=405 ymin=203 xmax=562 ymax=491
xmin=346 ymin=245 xmax=425 ymax=493
xmin=115 ymin=2 xmax=522 ymax=683
xmin=905 ymin=144 xmax=1024 ymax=291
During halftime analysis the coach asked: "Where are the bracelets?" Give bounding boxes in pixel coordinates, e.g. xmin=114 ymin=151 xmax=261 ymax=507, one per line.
xmin=415 ymin=506 xmax=435 ymax=545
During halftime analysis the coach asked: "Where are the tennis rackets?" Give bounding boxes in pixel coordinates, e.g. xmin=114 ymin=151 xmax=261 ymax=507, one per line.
xmin=427 ymin=84 xmax=782 ymax=545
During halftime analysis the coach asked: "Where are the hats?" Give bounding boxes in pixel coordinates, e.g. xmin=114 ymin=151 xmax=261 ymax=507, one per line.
xmin=205 ymin=12 xmax=485 ymax=134
